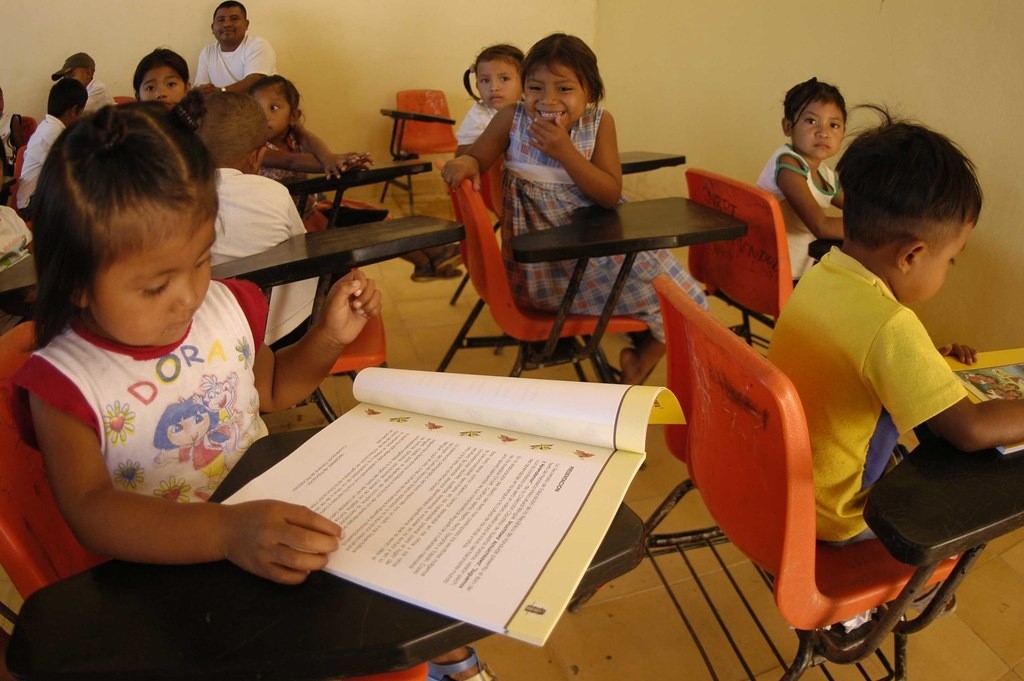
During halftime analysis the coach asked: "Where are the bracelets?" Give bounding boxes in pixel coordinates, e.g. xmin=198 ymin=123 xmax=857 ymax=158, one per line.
xmin=221 ymin=87 xmax=225 ymax=92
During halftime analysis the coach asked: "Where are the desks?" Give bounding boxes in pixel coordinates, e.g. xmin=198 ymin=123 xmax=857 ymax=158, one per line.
xmin=619 ymin=152 xmax=686 ymax=176
xmin=209 ymin=215 xmax=465 ymax=290
xmin=815 ymin=422 xmax=1024 ymax=664
xmin=380 ymin=108 xmax=456 ymax=160
xmin=508 ymin=196 xmax=748 ymax=365
xmin=6 ymin=425 xmax=648 ymax=681
xmin=809 ymin=238 xmax=844 ymax=261
xmin=1 ymin=175 xmax=38 ymax=309
xmin=282 ymin=158 xmax=432 ymax=231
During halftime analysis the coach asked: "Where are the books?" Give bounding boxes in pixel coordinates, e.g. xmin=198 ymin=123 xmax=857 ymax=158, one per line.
xmin=942 ymin=347 xmax=1024 ymax=456
xmin=219 ymin=367 xmax=689 ymax=649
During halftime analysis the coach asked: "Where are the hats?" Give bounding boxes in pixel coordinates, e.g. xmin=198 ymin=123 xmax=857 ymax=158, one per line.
xmin=50 ymin=52 xmax=96 ymax=81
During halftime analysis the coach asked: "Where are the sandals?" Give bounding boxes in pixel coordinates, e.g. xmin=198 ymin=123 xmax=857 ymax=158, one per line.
xmin=411 ymin=262 xmax=462 ymax=282
xmin=433 ymin=243 xmax=462 ymax=273
xmin=423 ymin=641 xmax=500 ymax=681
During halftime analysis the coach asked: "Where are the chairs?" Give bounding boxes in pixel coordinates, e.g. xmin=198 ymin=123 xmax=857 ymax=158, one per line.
xmin=450 ymin=150 xmax=505 ymax=306
xmin=0 ymin=321 xmax=430 ymax=681
xmin=686 ymin=167 xmax=795 ymax=358
xmin=568 ymin=274 xmax=964 ymax=681
xmin=260 ymin=313 xmax=389 ymax=430
xmin=433 ymin=157 xmax=650 ymax=385
xmin=379 ymin=89 xmax=458 ymax=218
xmin=9 ymin=95 xmax=136 ymax=235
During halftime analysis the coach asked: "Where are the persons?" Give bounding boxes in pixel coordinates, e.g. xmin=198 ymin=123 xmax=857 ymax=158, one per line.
xmin=453 ymin=45 xmax=526 ymax=161
xmin=0 ymin=46 xmax=316 ymax=352
xmin=439 ymin=34 xmax=709 ymax=387
xmin=756 ymin=76 xmax=848 ymax=294
xmin=190 ymin=0 xmax=275 ymax=96
xmin=248 ymin=73 xmax=464 ymax=285
xmin=12 ymin=100 xmax=497 ymax=681
xmin=768 ymin=119 xmax=1023 ymax=642
